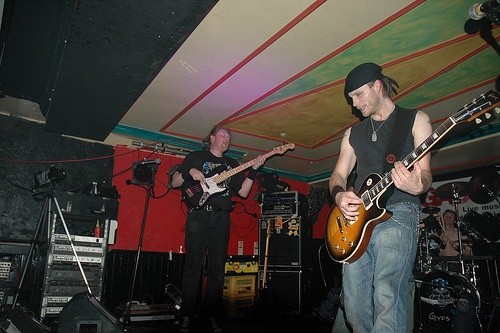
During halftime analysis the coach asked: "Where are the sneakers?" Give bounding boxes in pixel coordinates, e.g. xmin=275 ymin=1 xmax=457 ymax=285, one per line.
xmin=206 ymin=319 xmax=222 ymax=332
xmin=181 ymin=316 xmax=191 ymax=331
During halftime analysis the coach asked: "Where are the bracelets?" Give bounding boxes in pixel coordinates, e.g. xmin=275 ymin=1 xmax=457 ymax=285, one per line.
xmin=331 ymin=185 xmax=345 ymax=201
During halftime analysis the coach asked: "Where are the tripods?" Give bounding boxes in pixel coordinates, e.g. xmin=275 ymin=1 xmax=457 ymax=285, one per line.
xmin=11 ymin=185 xmax=92 ymax=310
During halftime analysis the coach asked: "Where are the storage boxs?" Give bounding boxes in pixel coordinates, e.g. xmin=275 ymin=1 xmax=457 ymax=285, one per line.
xmin=261 ymin=191 xmax=308 ymax=218
xmin=224 ymin=276 xmax=256 ymax=305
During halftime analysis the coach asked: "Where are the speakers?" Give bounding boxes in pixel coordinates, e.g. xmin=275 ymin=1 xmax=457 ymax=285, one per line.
xmin=0 ymin=291 xmax=124 ymax=333
xmin=257 ymin=217 xmax=310 ymax=316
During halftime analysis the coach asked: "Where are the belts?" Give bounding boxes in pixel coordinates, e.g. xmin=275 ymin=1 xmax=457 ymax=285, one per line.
xmin=201 ymin=205 xmax=218 ymax=211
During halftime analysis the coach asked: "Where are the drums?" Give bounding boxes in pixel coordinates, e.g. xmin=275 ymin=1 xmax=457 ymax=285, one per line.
xmin=411 ymin=273 xmax=481 ymax=331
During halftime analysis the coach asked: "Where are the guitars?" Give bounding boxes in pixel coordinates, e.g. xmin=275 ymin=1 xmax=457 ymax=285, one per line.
xmin=324 ymin=89 xmax=500 ymax=265
xmin=180 ymin=141 xmax=297 ymax=209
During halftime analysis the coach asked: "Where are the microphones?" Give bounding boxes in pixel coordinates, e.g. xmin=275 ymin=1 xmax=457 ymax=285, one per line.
xmin=469 ymin=0 xmax=500 ymax=20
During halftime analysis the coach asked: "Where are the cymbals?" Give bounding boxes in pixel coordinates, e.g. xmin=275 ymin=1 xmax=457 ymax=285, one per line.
xmin=436 ymin=181 xmax=473 ymax=197
xmin=469 ymin=170 xmax=500 ymax=204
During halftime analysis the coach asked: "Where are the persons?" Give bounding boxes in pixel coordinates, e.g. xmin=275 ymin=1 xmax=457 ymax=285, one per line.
xmin=330 ymin=62 xmax=432 ymax=333
xmin=437 ymin=210 xmax=473 ymax=258
xmin=172 ymin=127 xmax=266 ymax=325
